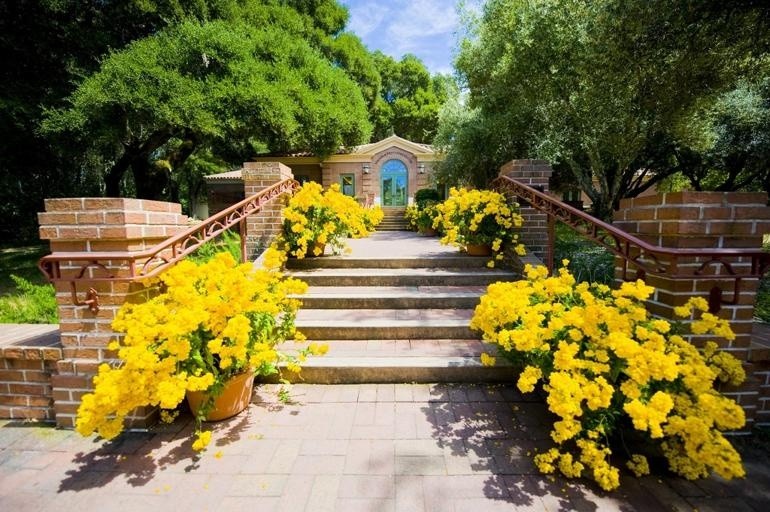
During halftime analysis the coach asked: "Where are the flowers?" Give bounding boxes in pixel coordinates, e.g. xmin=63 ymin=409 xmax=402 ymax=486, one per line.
xmin=404 ymin=199 xmax=436 ymax=233
xmin=433 ymin=187 xmax=525 ymax=268
xmin=470 ymin=258 xmax=746 ymax=492
xmin=271 ymin=182 xmax=385 ymax=258
xmin=73 ymin=249 xmax=329 ymax=451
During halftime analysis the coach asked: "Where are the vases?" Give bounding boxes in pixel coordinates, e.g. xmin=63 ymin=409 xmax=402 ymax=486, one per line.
xmin=422 ymin=227 xmax=435 ymax=237
xmin=306 ymin=242 xmax=324 ymax=257
xmin=186 ymin=373 xmax=253 ymax=421
xmin=467 ymin=243 xmax=490 ymax=255
